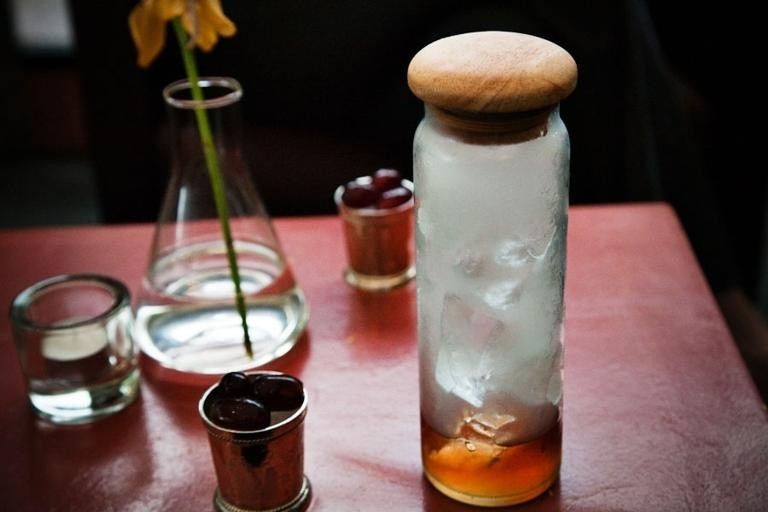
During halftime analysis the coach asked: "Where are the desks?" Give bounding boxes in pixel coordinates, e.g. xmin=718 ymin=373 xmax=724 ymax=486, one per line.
xmin=0 ymin=200 xmax=768 ymax=511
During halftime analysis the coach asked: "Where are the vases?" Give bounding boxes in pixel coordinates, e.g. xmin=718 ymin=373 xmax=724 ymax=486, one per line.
xmin=127 ymin=76 xmax=309 ymax=376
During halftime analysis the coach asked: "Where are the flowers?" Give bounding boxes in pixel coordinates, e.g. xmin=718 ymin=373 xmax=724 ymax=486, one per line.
xmin=128 ymin=0 xmax=252 ymax=357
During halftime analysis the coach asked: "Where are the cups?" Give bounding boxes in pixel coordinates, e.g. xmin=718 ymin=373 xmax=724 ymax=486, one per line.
xmin=198 ymin=368 xmax=317 ymax=512
xmin=10 ymin=273 xmax=140 ymax=424
xmin=331 ymin=174 xmax=417 ymax=291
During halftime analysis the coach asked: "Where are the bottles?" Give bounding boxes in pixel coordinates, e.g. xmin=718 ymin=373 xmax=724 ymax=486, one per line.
xmin=406 ymin=29 xmax=581 ymax=507
